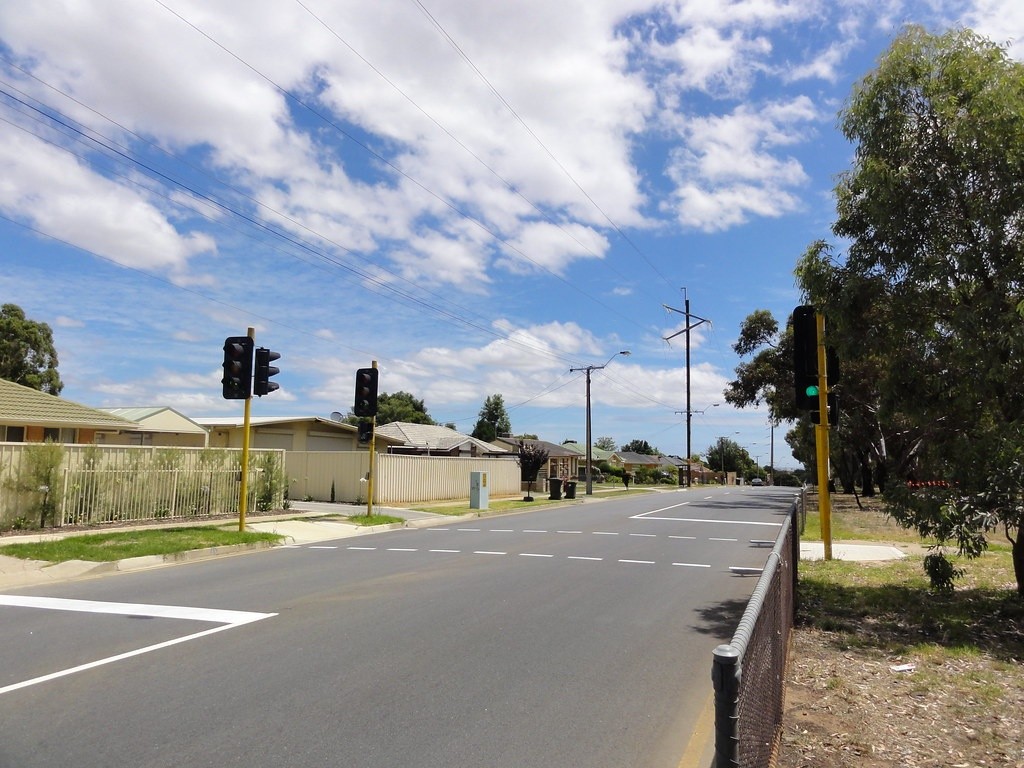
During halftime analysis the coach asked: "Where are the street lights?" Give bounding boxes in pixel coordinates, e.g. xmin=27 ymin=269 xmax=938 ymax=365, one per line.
xmin=586 ymin=351 xmax=631 ymax=495
xmin=721 ymin=432 xmax=740 ymax=486
xmin=687 ymin=404 xmax=719 ymax=487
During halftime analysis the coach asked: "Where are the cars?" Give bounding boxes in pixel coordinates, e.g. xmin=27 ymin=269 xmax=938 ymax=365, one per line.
xmin=752 ymin=478 xmax=762 ymax=486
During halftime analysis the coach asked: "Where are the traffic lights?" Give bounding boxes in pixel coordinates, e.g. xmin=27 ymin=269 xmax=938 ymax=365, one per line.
xmin=805 ymin=375 xmax=820 ymax=424
xmin=221 ymin=336 xmax=254 ymax=399
xmin=254 ymin=348 xmax=281 ymax=397
xmin=354 ymin=368 xmax=378 ymax=416
xmin=358 ymin=423 xmax=373 ymax=442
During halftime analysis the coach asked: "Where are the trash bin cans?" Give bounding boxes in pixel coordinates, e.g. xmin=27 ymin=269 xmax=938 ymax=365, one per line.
xmin=564 ymin=481 xmax=578 ymax=499
xmin=736 ymin=479 xmax=740 ymax=485
xmin=547 ymin=477 xmax=564 ymax=500
